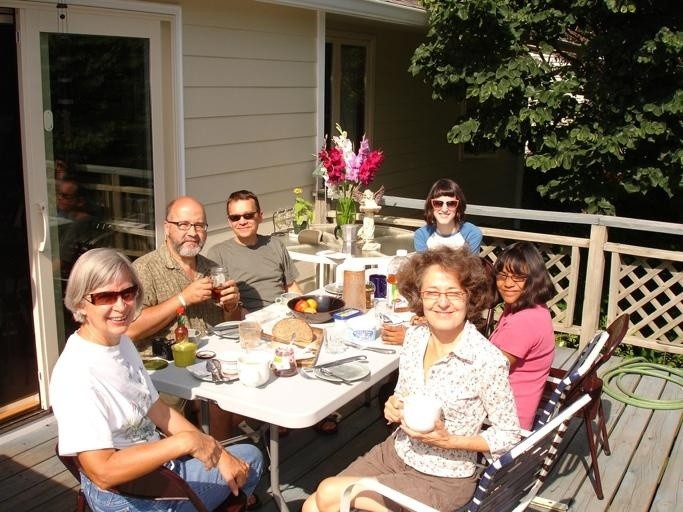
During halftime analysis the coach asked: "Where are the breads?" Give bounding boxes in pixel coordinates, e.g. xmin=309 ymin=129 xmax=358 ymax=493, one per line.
xmin=272 ymin=316 xmax=313 ymax=343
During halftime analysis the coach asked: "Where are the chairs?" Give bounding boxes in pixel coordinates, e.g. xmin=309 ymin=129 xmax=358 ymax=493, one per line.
xmin=482 ymin=331 xmax=611 ymax=439
xmin=55 ymin=441 xmax=247 ymax=512
xmin=339 ymin=394 xmax=592 ymax=512
xmin=528 ymin=312 xmax=629 ymax=500
xmin=474 ymin=255 xmax=498 ymax=338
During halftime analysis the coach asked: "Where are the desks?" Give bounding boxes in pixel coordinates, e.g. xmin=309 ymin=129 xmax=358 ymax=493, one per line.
xmin=272 ymin=223 xmax=416 ymax=288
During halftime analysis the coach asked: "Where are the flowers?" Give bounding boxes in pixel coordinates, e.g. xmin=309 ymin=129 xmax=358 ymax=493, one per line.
xmin=291 ymin=188 xmax=313 ymax=225
xmin=311 ymin=122 xmax=385 ymax=225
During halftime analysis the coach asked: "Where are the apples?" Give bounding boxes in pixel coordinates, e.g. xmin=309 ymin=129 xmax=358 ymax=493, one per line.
xmin=294 ymin=298 xmax=317 ymax=313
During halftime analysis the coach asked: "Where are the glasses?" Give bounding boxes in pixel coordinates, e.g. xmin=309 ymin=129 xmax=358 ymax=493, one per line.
xmin=430 ymin=199 xmax=460 ymax=210
xmin=418 ymin=290 xmax=469 ymax=299
xmin=498 ymin=273 xmax=526 ymax=282
xmin=227 ymin=212 xmax=258 ymax=222
xmin=81 ymin=282 xmax=141 ymax=305
xmin=166 ymin=220 xmax=209 ymax=232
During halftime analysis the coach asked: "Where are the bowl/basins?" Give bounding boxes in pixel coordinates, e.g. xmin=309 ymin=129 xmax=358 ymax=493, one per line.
xmin=286 ymin=294 xmax=346 ymax=324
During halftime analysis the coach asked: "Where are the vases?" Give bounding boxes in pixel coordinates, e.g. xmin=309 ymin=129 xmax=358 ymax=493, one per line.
xmin=336 ymin=198 xmax=357 ymax=237
xmin=293 ymin=221 xmax=306 ymax=234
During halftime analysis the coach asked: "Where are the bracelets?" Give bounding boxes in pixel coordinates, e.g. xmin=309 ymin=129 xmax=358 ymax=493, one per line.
xmin=176 ymin=293 xmax=187 ymax=312
xmin=222 ymin=303 xmax=239 ymax=315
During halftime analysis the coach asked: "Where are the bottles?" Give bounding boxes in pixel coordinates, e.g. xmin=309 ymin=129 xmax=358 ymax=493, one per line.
xmin=363 ymin=263 xmax=380 ymax=285
xmin=385 ymin=249 xmax=408 ymax=310
xmin=209 ymin=265 xmax=226 ymax=303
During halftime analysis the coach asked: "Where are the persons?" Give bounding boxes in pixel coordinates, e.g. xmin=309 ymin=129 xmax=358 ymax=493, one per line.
xmin=55 ymin=150 xmax=99 ymax=270
xmin=47 ymin=246 xmax=265 ymax=512
xmin=301 ymin=243 xmax=522 ymax=512
xmin=487 ymin=239 xmax=556 ymax=440
xmin=207 ymin=190 xmax=304 ymax=316
xmin=125 ymin=196 xmax=242 ymax=359
xmin=414 ymin=177 xmax=483 ymax=255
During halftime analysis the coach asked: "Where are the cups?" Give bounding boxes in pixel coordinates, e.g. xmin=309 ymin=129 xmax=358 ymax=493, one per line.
xmin=368 ymin=274 xmax=386 ymax=300
xmin=401 ymin=394 xmax=443 ymax=434
xmin=333 ymin=223 xmax=357 ymax=242
xmin=274 ymin=291 xmax=302 ymax=321
xmin=365 ymin=287 xmax=374 ymax=308
xmin=342 ymin=268 xmax=366 ymax=314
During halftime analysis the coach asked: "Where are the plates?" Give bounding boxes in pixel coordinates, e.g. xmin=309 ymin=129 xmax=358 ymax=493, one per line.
xmin=324 ymin=283 xmax=344 ymax=294
xmin=142 ymin=306 xmax=410 ymax=389
